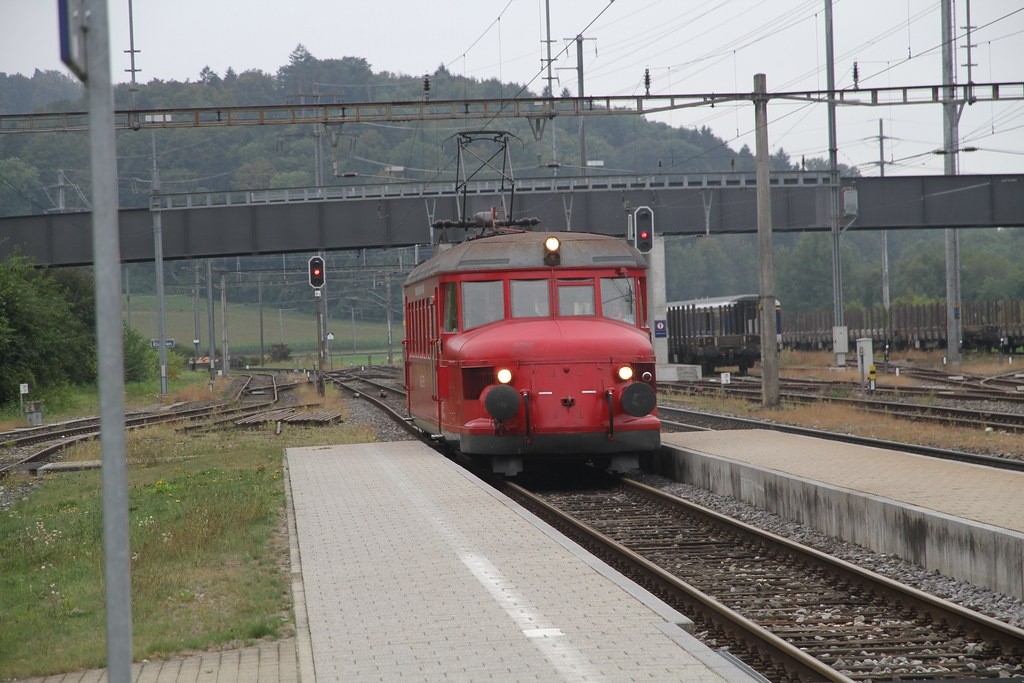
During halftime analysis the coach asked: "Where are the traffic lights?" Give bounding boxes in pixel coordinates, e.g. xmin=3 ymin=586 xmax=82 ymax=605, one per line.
xmin=308 ymin=256 xmax=325 ymax=288
xmin=633 ymin=205 xmax=654 ymax=255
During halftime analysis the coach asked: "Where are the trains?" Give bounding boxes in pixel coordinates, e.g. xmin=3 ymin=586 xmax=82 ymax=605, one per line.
xmin=664 ymin=292 xmax=782 ymax=374
xmin=403 ymin=231 xmax=662 ymax=473
